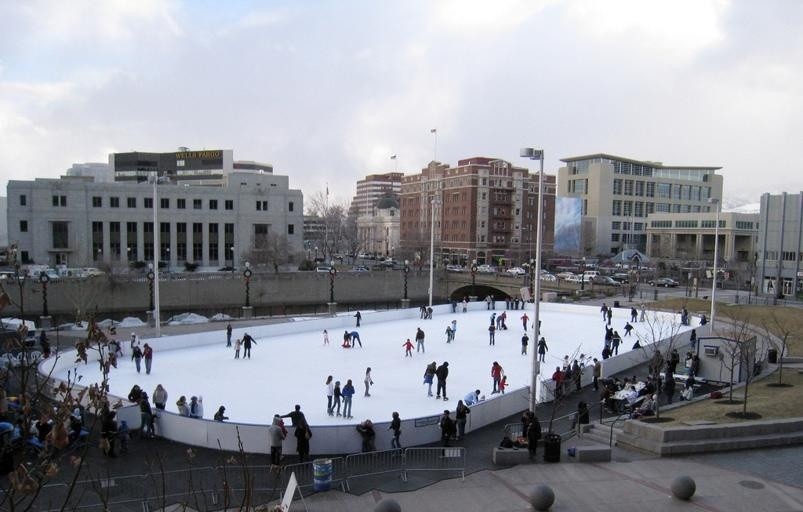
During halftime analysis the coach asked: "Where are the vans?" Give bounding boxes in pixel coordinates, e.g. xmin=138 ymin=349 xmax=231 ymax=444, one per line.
xmin=30 ymin=268 xmax=58 ymax=280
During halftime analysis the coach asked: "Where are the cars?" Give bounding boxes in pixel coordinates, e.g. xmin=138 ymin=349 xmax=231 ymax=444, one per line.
xmin=565 ymin=274 xmax=589 ymax=283
xmin=647 ymin=278 xmax=679 ymax=287
xmin=335 ymin=254 xmax=342 ymax=260
xmin=371 ymin=263 xmax=381 ymax=270
xmin=540 ymin=269 xmax=555 ymax=283
xmin=353 ymin=266 xmax=367 ymax=271
xmin=344 ymin=250 xmax=385 ymax=261
xmin=555 ymin=272 xmax=573 ymax=278
xmin=391 ymin=246 xmax=394 ymax=255
xmin=380 ymin=261 xmax=393 ymax=267
xmin=447 ymin=264 xmax=464 ymax=273
xmin=578 ymin=271 xmax=600 ymax=280
xmin=61 ymin=268 xmax=86 ymax=279
xmin=83 ymin=268 xmax=106 ymax=278
xmin=507 ymin=267 xmax=525 ymax=275
xmin=314 ymin=257 xmax=333 ymax=265
xmin=590 ymin=276 xmax=619 ymax=287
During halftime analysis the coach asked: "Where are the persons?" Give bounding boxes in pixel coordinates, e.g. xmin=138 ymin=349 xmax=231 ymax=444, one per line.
xmin=424 ymin=361 xmax=449 ymax=400
xmin=680 ymin=306 xmax=706 ymax=325
xmin=324 ymin=375 xmax=355 ymax=418
xmin=491 ymin=361 xmax=509 ymax=394
xmin=357 ymin=419 xmax=376 ymax=452
xmin=364 ymin=368 xmax=374 ymax=396
xmin=521 ymin=312 xmax=549 ymax=362
xmin=649 ymin=329 xmax=700 ymax=404
xmin=2 ymin=402 xmax=85 ymax=473
xmin=403 ymin=328 xmax=425 ymax=357
xmin=101 ymin=383 xmax=168 ymax=457
xmin=100 ymin=333 xmax=153 ymax=375
xmin=552 ymin=352 xmax=656 ymax=429
xmin=520 ymin=410 xmax=541 ymax=456
xmin=388 ymin=412 xmax=403 ymax=455
xmin=39 ymin=330 xmax=51 ymax=358
xmin=445 ymin=320 xmax=456 ymax=343
xmin=269 ymin=405 xmax=312 ymax=464
xmin=177 ymin=395 xmax=204 ymax=418
xmin=323 ymin=311 xmax=363 ymax=348
xmin=601 ymin=302 xmax=645 ymax=359
xmin=453 ymin=297 xmax=467 ymax=313
xmin=420 ymin=305 xmax=433 ymax=319
xmin=506 ymin=296 xmax=524 ymax=310
xmin=486 ymin=295 xmax=495 ymax=310
xmin=440 ymin=390 xmax=485 ymax=457
xmin=488 ymin=311 xmax=506 ymax=345
xmin=226 ymin=324 xmax=257 ymax=359
xmin=214 ymin=406 xmax=228 ymax=422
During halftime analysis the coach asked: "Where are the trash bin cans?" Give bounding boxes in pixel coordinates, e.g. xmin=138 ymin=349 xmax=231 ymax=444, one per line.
xmin=769 ymin=349 xmax=777 ymax=363
xmin=614 ymin=301 xmax=619 ymax=307
xmin=313 ymin=458 xmax=332 ymax=492
xmin=545 ymin=434 xmax=562 ymax=463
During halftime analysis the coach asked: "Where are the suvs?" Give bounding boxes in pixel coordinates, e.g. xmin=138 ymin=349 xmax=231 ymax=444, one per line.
xmin=314 ymin=267 xmax=338 ymax=272
xmin=610 ymin=273 xmax=629 ymax=284
xmin=0 ymin=250 xmax=8 ymax=263
xmin=0 ymin=272 xmax=24 ymax=281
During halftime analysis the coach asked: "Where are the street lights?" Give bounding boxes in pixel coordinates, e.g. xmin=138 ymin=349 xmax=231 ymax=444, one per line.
xmin=581 ymin=256 xmax=586 ymax=290
xmin=126 ymin=248 xmax=131 ymax=263
xmin=470 ymin=260 xmax=476 ymax=296
xmin=166 ymin=248 xmax=170 ymax=272
xmin=328 ymin=261 xmax=335 ymax=302
xmin=315 ymin=247 xmax=318 ymax=258
xmin=146 ymin=263 xmax=154 ymax=311
xmin=242 ymin=262 xmax=251 ymax=306
xmin=708 ymin=198 xmax=719 ymax=334
xmin=37 ymin=267 xmax=49 ymax=317
xmin=230 ymin=247 xmax=235 ymax=278
xmin=402 ymin=260 xmax=409 ymax=298
xmin=306 ymin=240 xmax=310 ymax=258
xmin=428 ymin=200 xmax=435 ymax=305
xmin=147 ymin=174 xmax=161 ymax=339
xmin=97 ymin=248 xmax=102 ymax=262
xmin=518 ymin=148 xmax=544 ymax=412
xmin=529 ymin=259 xmax=534 ymax=298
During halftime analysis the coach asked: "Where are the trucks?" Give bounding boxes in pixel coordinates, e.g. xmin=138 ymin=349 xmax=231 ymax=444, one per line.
xmin=477 ymin=265 xmax=498 ymax=272
xmin=0 ymin=316 xmax=35 ymax=352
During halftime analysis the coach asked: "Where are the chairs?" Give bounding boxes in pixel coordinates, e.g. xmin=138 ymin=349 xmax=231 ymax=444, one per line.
xmin=0 ymin=345 xmax=93 ymax=472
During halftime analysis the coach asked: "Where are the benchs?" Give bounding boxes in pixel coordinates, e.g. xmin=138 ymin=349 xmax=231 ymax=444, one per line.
xmin=598 ymin=370 xmax=707 ymax=417
xmin=492 ymin=446 xmax=534 ymax=464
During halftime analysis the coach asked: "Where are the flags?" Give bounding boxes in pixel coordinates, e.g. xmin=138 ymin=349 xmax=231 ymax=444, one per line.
xmin=430 ymin=129 xmax=436 ymax=134
xmin=390 ymin=155 xmax=396 ymax=161
